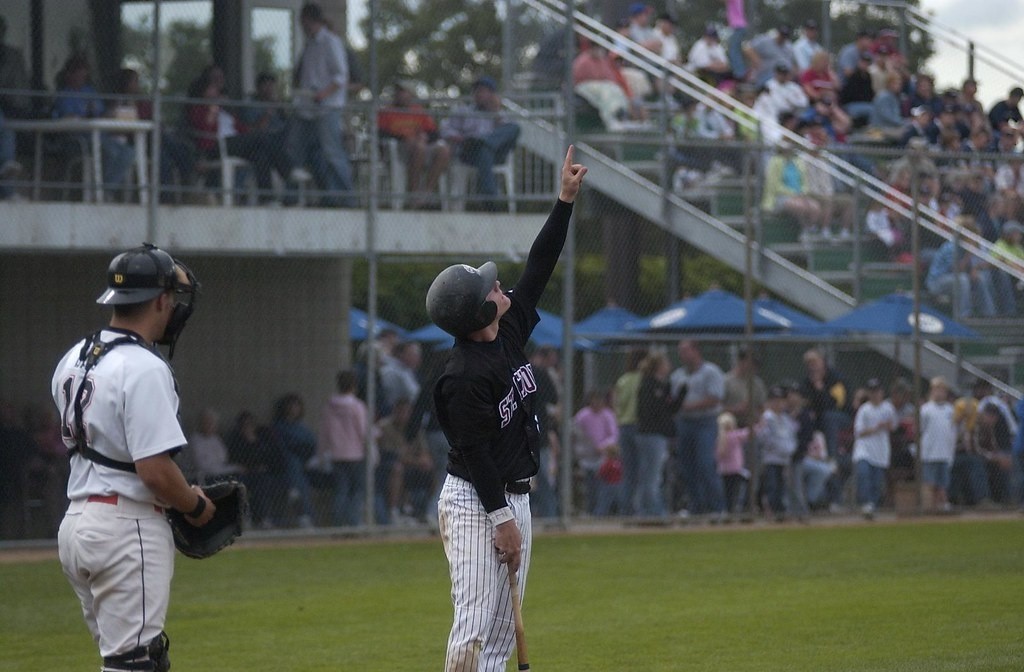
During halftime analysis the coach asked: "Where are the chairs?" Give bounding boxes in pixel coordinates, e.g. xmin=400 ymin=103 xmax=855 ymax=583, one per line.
xmin=164 ymin=112 xmax=518 ymax=215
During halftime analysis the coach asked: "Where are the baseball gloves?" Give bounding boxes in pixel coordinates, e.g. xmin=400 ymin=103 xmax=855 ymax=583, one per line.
xmin=167 ymin=478 xmax=252 ymax=560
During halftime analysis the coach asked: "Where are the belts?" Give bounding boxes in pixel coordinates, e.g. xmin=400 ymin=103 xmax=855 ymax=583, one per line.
xmin=88 ymin=494 xmax=162 ymax=515
xmin=506 ymin=481 xmax=531 ymax=495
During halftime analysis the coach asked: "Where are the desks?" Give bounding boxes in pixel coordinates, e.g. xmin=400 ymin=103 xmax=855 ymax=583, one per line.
xmin=0 ymin=118 xmax=153 ymax=205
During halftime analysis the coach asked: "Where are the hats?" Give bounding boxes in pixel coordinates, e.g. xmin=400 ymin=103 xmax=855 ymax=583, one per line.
xmin=397 ymin=81 xmax=419 ymax=98
xmin=469 ymin=79 xmax=498 ymax=90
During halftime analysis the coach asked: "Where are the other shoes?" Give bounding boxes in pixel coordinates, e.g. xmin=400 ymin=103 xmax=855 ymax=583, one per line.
xmin=858 ymin=501 xmax=876 ymax=518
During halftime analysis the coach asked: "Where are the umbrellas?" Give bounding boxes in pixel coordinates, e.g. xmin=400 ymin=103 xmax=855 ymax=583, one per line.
xmin=528 ymin=289 xmax=978 ymax=352
xmin=350 ymin=306 xmax=455 ymax=351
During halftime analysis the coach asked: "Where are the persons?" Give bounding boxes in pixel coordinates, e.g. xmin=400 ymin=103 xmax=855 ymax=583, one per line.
xmin=51 ymin=242 xmax=247 ymax=672
xmin=532 ymin=338 xmax=1024 ymax=517
xmin=0 ymin=1 xmax=520 ymax=213
xmin=570 ymin=0 xmax=1024 ymax=318
xmin=186 ymin=329 xmax=449 ymax=528
xmin=426 ymin=144 xmax=588 ymax=672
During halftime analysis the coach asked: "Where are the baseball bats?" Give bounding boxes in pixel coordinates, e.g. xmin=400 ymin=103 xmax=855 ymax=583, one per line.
xmin=505 ymin=551 xmax=534 ymax=672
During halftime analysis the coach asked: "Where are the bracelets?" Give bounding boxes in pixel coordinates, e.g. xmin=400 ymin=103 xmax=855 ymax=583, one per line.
xmin=189 ymin=495 xmax=206 ymax=519
xmin=488 ymin=506 xmax=515 ymax=526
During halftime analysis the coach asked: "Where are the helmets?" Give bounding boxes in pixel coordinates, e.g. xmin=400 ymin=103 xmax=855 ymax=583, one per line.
xmin=425 ymin=261 xmax=497 ymax=336
xmin=96 ymin=242 xmax=176 ymax=302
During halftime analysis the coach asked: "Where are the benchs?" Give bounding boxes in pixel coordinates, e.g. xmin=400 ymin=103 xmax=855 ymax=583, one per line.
xmin=529 ymin=110 xmax=1024 ymax=390
